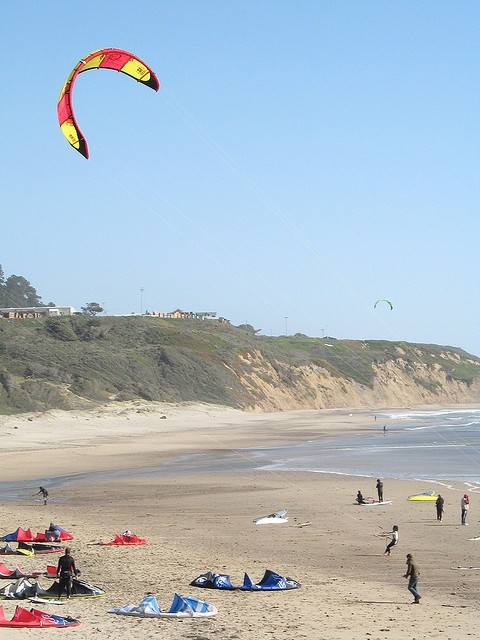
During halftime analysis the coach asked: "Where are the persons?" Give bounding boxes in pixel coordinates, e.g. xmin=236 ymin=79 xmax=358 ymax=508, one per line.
xmin=56 ymin=547 xmax=77 ymax=599
xmin=461 ymin=494 xmax=469 ymax=526
xmin=435 ymin=494 xmax=444 ymax=522
xmin=376 ymin=479 xmax=384 ymax=502
xmin=356 ymin=490 xmax=366 ymax=504
xmin=401 ymin=554 xmax=422 ymax=604
xmin=378 ymin=526 xmax=399 ymax=556
xmin=32 ymin=487 xmax=48 ymax=497
